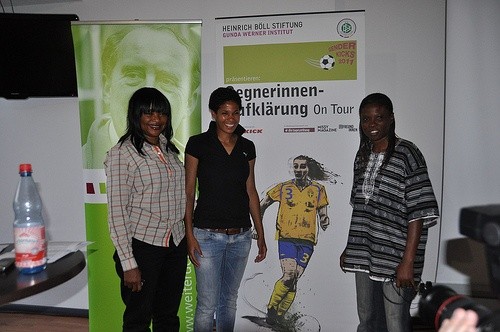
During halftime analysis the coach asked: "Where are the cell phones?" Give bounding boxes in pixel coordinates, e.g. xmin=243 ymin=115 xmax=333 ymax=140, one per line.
xmin=0 ymin=259 xmax=16 ymax=272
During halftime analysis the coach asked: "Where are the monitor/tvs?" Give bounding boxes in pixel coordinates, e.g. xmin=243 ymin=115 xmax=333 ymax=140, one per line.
xmin=0 ymin=13 xmax=79 ymax=99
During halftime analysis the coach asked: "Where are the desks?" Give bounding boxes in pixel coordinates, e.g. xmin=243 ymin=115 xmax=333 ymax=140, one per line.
xmin=0 ymin=242 xmax=85 ymax=305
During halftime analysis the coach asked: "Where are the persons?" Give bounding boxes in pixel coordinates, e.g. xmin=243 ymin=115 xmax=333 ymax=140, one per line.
xmin=437 ymin=307 xmax=480 ymax=332
xmin=104 ymin=87 xmax=191 ymax=332
xmin=78 ymin=23 xmax=202 ymax=171
xmin=184 ymin=87 xmax=268 ymax=331
xmin=340 ymin=92 xmax=440 ymax=332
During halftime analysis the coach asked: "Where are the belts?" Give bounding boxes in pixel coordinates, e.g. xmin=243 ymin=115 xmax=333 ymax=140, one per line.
xmin=203 ymin=225 xmax=251 ymax=235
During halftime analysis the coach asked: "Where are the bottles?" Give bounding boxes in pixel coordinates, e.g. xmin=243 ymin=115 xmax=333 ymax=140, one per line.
xmin=12 ymin=164 xmax=47 ymax=274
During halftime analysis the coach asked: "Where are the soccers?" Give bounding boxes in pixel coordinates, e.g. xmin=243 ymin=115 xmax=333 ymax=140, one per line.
xmin=319 ymin=54 xmax=336 ymax=71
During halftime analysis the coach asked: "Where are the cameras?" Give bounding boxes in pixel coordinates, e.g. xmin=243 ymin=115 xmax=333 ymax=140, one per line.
xmin=418 ymin=285 xmax=500 ymax=332
